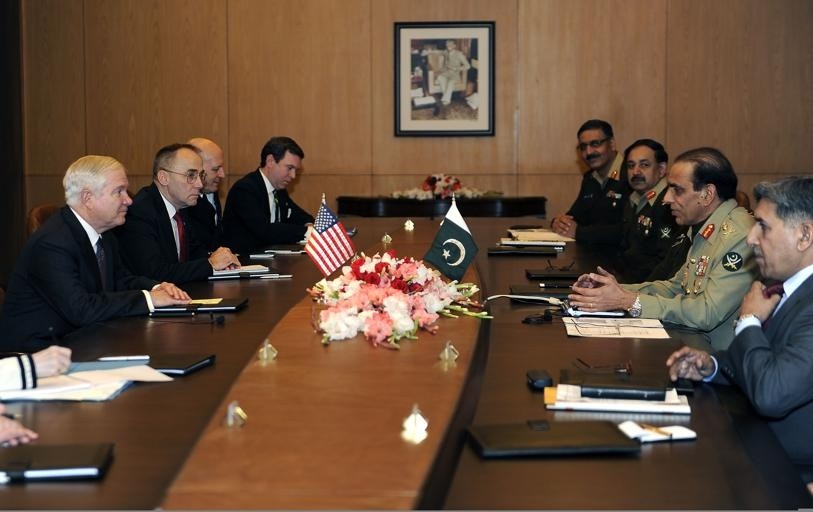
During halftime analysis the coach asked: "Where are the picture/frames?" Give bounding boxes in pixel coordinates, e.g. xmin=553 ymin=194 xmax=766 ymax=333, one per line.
xmin=391 ymin=19 xmax=496 ymax=138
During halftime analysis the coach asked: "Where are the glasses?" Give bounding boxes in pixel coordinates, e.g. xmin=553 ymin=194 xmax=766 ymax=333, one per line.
xmin=159 ymin=167 xmax=208 ymax=185
xmin=575 ymin=356 xmax=634 ymax=378
xmin=576 ymin=136 xmax=611 ymax=152
xmin=544 ymin=259 xmax=576 ymax=272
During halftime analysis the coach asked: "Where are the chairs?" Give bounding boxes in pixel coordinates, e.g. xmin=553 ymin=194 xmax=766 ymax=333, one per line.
xmin=424 ymin=49 xmax=468 ymax=97
xmin=26 ymin=202 xmax=62 ymax=238
xmin=734 ymin=189 xmax=751 ymax=212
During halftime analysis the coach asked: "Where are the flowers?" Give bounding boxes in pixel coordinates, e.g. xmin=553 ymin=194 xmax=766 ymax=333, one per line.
xmin=302 ymin=244 xmax=496 ymax=349
xmin=377 ymin=169 xmax=506 ymax=201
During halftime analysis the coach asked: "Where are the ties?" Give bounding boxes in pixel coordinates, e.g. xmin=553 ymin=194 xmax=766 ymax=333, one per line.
xmin=172 ymin=211 xmax=187 ymax=263
xmin=762 ymin=282 xmax=786 ymax=334
xmin=272 ymin=188 xmax=282 ymax=223
xmin=95 ymin=236 xmax=107 ymax=294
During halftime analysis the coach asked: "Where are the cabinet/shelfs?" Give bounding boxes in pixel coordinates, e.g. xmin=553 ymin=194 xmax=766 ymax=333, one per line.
xmin=334 ymin=193 xmax=550 ymax=219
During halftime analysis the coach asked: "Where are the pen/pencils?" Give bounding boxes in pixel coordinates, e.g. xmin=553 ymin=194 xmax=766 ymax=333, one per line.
xmin=207 ymin=252 xmax=240 ymax=256
xmin=149 ymin=312 xmax=196 ymax=318
xmin=642 ymin=424 xmax=673 ymax=437
xmin=48 ymin=327 xmax=60 ymax=346
xmin=539 ymin=283 xmax=573 ymax=288
xmin=1 ymin=413 xmax=23 ymax=419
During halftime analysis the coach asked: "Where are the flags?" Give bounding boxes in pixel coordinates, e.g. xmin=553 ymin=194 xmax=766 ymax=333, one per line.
xmin=422 ymin=201 xmax=480 ymax=285
xmin=305 ymin=201 xmax=356 ymax=281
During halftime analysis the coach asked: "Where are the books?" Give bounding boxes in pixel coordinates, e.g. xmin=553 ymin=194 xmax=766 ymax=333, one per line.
xmin=150 ymin=298 xmax=248 ymax=311
xmin=463 ymin=368 xmax=696 ymax=460
xmin=510 ymin=267 xmax=625 ymax=318
xmin=487 ymin=224 xmax=577 ymax=257
xmin=212 ymin=248 xmax=305 ymax=280
xmin=0 ymin=353 xmax=215 ymax=400
xmin=0 ymin=440 xmax=117 ymax=483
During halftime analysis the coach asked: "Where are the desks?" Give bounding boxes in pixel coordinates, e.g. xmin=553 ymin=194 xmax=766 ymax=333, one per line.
xmin=0 ymin=219 xmax=812 ymax=511
xmin=410 ymin=95 xmax=441 ymax=117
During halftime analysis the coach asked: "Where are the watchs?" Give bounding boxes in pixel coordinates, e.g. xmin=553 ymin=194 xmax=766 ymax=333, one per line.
xmin=627 ymin=291 xmax=642 ymax=320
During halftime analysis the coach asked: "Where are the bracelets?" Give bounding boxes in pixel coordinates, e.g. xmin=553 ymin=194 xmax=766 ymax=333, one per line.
xmin=733 ymin=314 xmax=761 ymax=329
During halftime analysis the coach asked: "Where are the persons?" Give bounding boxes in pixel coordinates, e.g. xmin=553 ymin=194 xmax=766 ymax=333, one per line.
xmin=0 ymin=403 xmax=39 ymax=444
xmin=111 ymin=145 xmax=242 ymax=283
xmin=179 ymin=139 xmax=222 ymax=254
xmin=0 ymin=155 xmax=192 ymax=355
xmin=568 ymin=148 xmax=773 ymax=331
xmin=615 ymin=139 xmax=693 ymax=283
xmin=0 ymin=344 xmax=72 ymax=392
xmin=223 ymin=137 xmax=316 ymax=251
xmin=551 ymin=120 xmax=631 ymax=245
xmin=436 ymin=39 xmax=472 ymax=106
xmin=667 ymin=176 xmax=813 ymax=485
